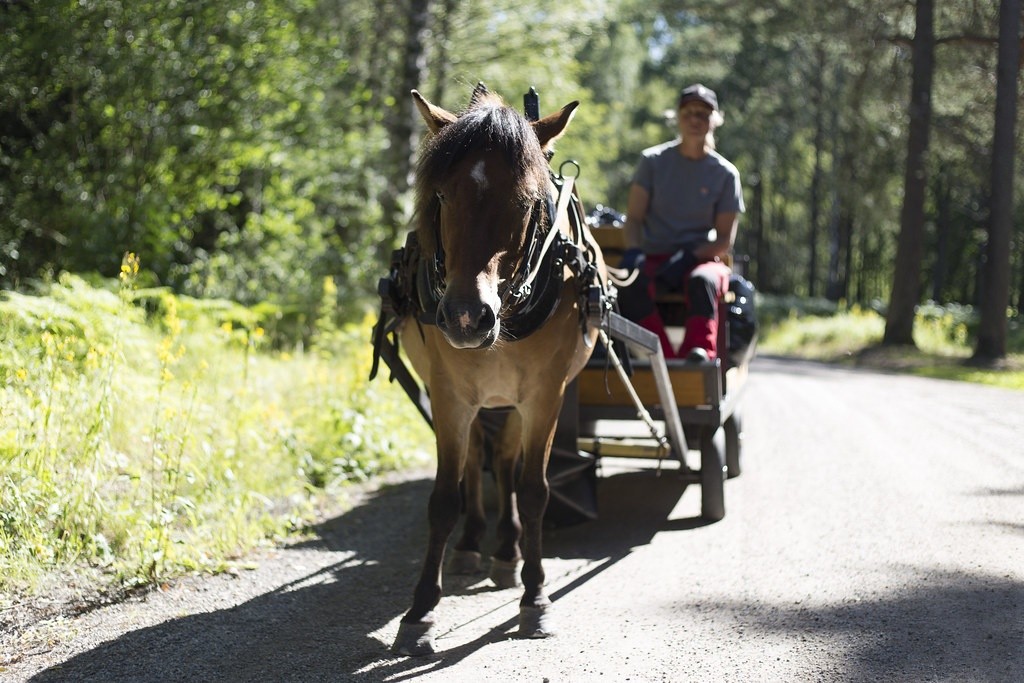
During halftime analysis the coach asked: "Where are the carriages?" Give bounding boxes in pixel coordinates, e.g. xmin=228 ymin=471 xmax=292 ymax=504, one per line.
xmin=368 ymin=81 xmax=746 ymax=659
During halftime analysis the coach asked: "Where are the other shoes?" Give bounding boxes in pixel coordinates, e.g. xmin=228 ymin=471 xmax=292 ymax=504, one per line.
xmin=626 ymin=344 xmax=648 ymax=358
xmin=688 ymin=347 xmax=709 ymax=360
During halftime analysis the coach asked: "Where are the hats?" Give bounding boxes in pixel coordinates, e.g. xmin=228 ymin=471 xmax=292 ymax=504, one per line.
xmin=680 ymin=83 xmax=718 ymax=110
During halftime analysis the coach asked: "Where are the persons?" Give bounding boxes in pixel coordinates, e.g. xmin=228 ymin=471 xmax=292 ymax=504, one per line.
xmin=617 ymin=83 xmax=746 ymax=363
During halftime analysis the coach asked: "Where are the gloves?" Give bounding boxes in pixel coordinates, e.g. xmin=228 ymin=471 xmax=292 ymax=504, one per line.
xmin=660 ymin=250 xmax=699 ymax=288
xmin=619 ymin=249 xmax=646 ymax=274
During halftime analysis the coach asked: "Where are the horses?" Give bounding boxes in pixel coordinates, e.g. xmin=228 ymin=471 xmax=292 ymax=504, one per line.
xmin=390 ymin=78 xmax=611 ymax=657
